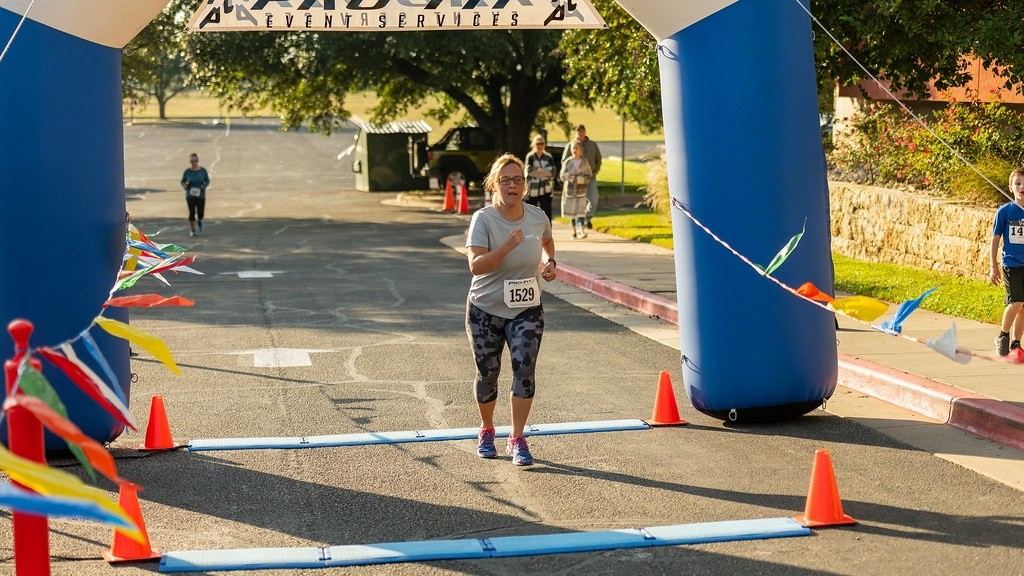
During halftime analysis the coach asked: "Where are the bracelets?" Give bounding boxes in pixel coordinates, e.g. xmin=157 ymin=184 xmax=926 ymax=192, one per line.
xmin=548 ymin=258 xmax=556 ymax=269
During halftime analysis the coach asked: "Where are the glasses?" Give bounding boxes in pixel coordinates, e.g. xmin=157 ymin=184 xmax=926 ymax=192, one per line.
xmin=496 ymin=176 xmax=525 ymax=185
xmin=190 ymin=160 xmax=198 ymax=163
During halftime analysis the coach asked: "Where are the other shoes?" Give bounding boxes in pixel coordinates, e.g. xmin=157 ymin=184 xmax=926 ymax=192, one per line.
xmin=190 ymin=230 xmax=196 ymax=236
xmin=580 ymin=231 xmax=588 ymax=238
xmin=198 ymin=221 xmax=202 ymax=232
xmin=571 ymin=234 xmax=576 ymax=239
xmin=587 ymin=223 xmax=593 ymax=228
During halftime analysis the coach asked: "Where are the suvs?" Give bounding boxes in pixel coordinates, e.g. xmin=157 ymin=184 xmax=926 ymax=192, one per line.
xmin=426 ymin=123 xmax=569 ymax=186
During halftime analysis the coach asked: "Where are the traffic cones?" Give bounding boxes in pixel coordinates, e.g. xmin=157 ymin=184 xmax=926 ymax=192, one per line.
xmin=641 ymin=371 xmax=692 ymax=427
xmin=101 ymin=480 xmax=165 ymax=565
xmin=793 ymin=448 xmax=860 ymax=532
xmin=457 ymin=182 xmax=473 ymax=214
xmin=144 ymin=394 xmax=185 ymax=452
xmin=441 ymin=177 xmax=462 ymax=215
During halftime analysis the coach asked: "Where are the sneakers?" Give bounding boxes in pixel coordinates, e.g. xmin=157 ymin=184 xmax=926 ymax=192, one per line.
xmin=1010 ymin=340 xmax=1024 ymax=352
xmin=477 ymin=426 xmax=498 ymax=459
xmin=506 ymin=434 xmax=534 ymax=466
xmin=994 ymin=335 xmax=1010 ymax=357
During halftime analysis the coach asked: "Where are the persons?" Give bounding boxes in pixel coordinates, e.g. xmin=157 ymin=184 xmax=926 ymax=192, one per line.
xmin=561 ymin=124 xmax=602 ymax=233
xmin=559 ymin=139 xmax=593 ymax=239
xmin=524 ymin=136 xmax=556 ymax=227
xmin=181 ymin=153 xmax=210 ymax=237
xmin=464 ymin=155 xmax=557 ymax=467
xmin=988 ymin=168 xmax=1024 ymax=356
